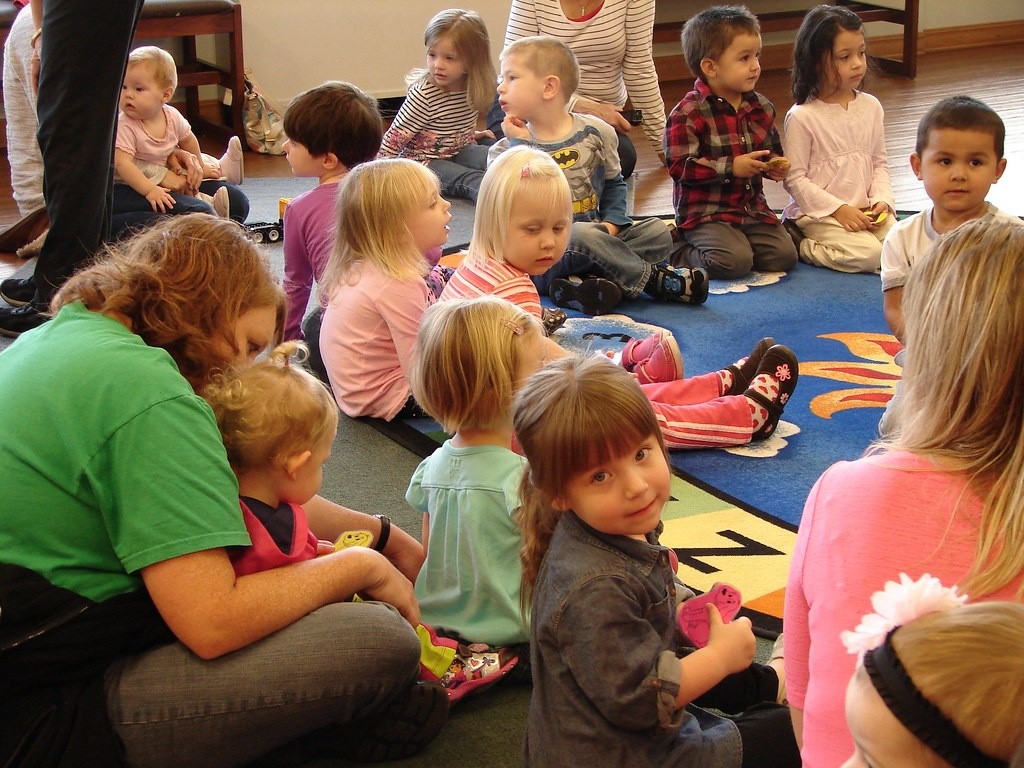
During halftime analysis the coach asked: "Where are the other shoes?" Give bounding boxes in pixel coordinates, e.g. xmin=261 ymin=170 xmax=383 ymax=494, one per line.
xmin=633 ymin=336 xmax=684 ymax=385
xmin=295 ymin=681 xmax=450 ymax=763
xmin=781 ymin=217 xmax=807 ymax=262
xmin=621 ymin=330 xmax=674 ymax=372
xmin=0 ymin=203 xmax=52 ymax=259
xmin=722 ymin=337 xmax=776 ymax=395
xmin=742 ymin=343 xmax=799 ymax=440
xmin=217 ymin=135 xmax=244 ymax=187
xmin=667 ymin=223 xmax=685 ymax=246
xmin=765 ymin=632 xmax=789 ymax=705
xmin=213 ymin=186 xmax=229 ymax=221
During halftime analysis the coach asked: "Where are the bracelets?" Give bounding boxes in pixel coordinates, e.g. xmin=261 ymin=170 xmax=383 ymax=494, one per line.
xmin=370 ymin=513 xmax=391 ymax=552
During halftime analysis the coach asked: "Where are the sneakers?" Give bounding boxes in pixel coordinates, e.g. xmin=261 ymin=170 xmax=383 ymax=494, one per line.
xmin=548 ymin=272 xmax=622 ymax=315
xmin=0 ymin=306 xmax=52 ymax=339
xmin=438 ymin=645 xmax=518 ymax=704
xmin=0 ymin=278 xmax=33 ymax=307
xmin=650 ymin=262 xmax=709 ymax=306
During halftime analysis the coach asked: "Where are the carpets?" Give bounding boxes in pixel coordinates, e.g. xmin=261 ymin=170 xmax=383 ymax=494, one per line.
xmin=355 ymin=210 xmax=922 ymax=640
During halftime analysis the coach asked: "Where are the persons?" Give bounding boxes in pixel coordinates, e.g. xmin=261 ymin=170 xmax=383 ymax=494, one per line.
xmin=1 ymin=0 xmax=251 ymax=339
xmin=271 ymin=2 xmax=1024 ymax=767
xmin=0 ymin=214 xmax=527 ymax=767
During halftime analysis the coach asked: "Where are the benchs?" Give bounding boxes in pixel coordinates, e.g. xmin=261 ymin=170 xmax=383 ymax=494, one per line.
xmin=0 ymin=0 xmax=248 ymax=147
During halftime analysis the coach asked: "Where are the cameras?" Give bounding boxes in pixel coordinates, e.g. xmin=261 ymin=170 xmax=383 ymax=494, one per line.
xmin=617 ymin=110 xmax=643 ymax=127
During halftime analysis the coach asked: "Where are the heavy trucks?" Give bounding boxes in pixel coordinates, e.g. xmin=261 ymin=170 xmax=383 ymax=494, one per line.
xmin=242 ymin=197 xmax=294 ymax=244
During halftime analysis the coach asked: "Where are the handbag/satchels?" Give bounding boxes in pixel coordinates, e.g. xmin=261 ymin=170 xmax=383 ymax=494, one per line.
xmin=220 ymin=68 xmax=292 ymax=156
